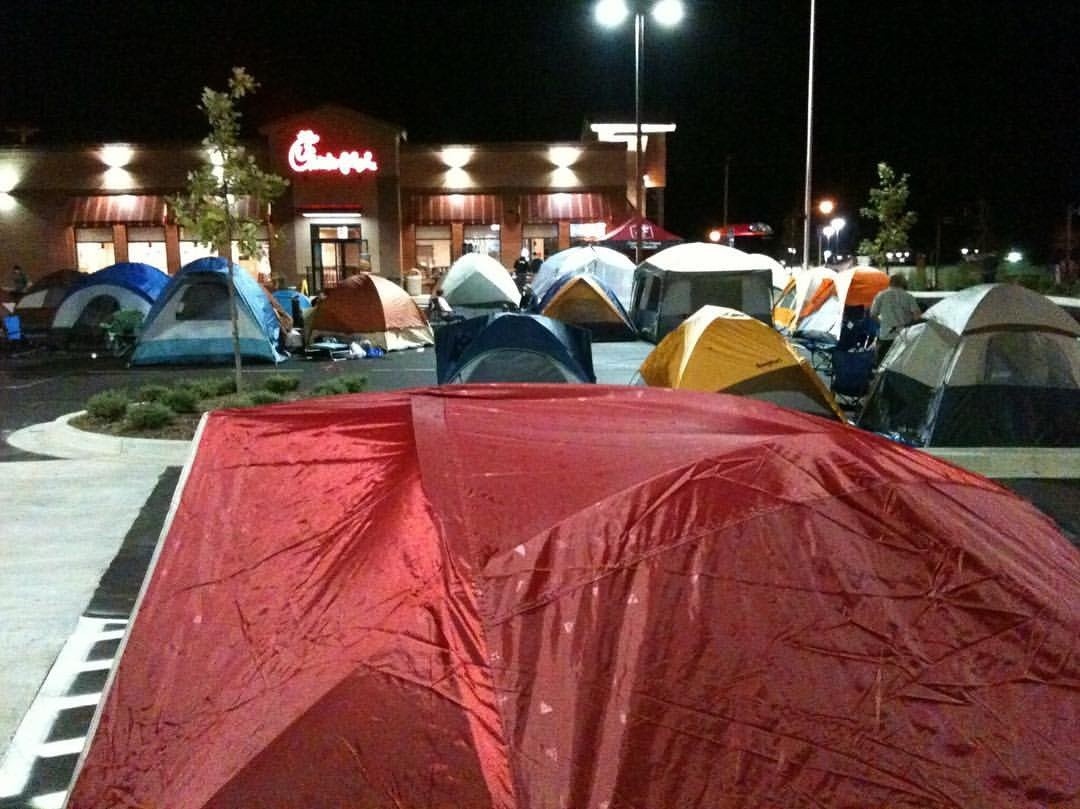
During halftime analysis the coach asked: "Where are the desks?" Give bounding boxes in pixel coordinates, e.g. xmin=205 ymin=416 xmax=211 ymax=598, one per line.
xmin=310 ymin=341 xmax=350 ymax=362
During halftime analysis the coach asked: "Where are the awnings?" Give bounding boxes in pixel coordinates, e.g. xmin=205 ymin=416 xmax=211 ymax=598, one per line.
xmin=71 ymin=195 xmax=167 ymax=228
xmin=408 ymin=194 xmax=504 ymax=225
xmin=528 ymin=193 xmax=612 ymax=224
xmin=178 ymin=193 xmax=271 ymax=226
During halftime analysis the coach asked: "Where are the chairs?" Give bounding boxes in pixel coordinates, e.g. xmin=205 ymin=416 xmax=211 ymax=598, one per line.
xmin=0 ymin=315 xmax=37 ymax=370
xmin=775 ymin=308 xmax=878 ymax=416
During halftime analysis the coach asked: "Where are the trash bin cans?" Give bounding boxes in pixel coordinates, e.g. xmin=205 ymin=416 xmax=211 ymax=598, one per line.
xmin=966 ymin=253 xmax=999 ymax=286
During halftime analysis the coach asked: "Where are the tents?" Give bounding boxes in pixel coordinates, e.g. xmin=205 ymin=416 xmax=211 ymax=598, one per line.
xmin=627 ymin=242 xmax=772 ymax=343
xmin=524 ymin=245 xmax=637 ymax=341
xmin=48 ymin=262 xmax=171 ymax=346
xmin=858 ymin=283 xmax=1080 ymax=448
xmin=631 ymin=303 xmax=848 ymax=423
xmin=438 ymin=252 xmax=522 ymax=318
xmin=129 ymin=257 xmax=288 ymax=364
xmin=13 ymin=269 xmax=87 ymax=339
xmin=309 ymin=274 xmax=434 ymax=352
xmin=596 ymin=215 xmax=683 ymax=250
xmin=432 ymin=313 xmax=596 ymax=385
xmin=69 ymin=384 xmax=1080 ymax=809
xmin=773 ymin=266 xmax=890 ymax=341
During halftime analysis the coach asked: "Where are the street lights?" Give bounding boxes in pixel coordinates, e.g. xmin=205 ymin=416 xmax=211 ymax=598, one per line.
xmin=824 ymin=226 xmax=835 ymax=250
xmin=831 ymin=218 xmax=846 ymax=257
xmin=593 ymin=0 xmax=685 ymax=267
xmin=787 ymin=248 xmax=797 ymax=270
xmin=823 ymin=250 xmax=832 ymax=266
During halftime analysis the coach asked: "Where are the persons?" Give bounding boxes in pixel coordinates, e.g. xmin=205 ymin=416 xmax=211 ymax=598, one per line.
xmin=870 ymin=274 xmax=920 ymax=366
xmin=431 ymin=290 xmax=446 ymax=320
xmin=13 ymin=266 xmax=28 ymax=289
xmin=531 ymin=251 xmax=543 ymax=274
xmin=514 ymin=254 xmax=528 ymax=287
